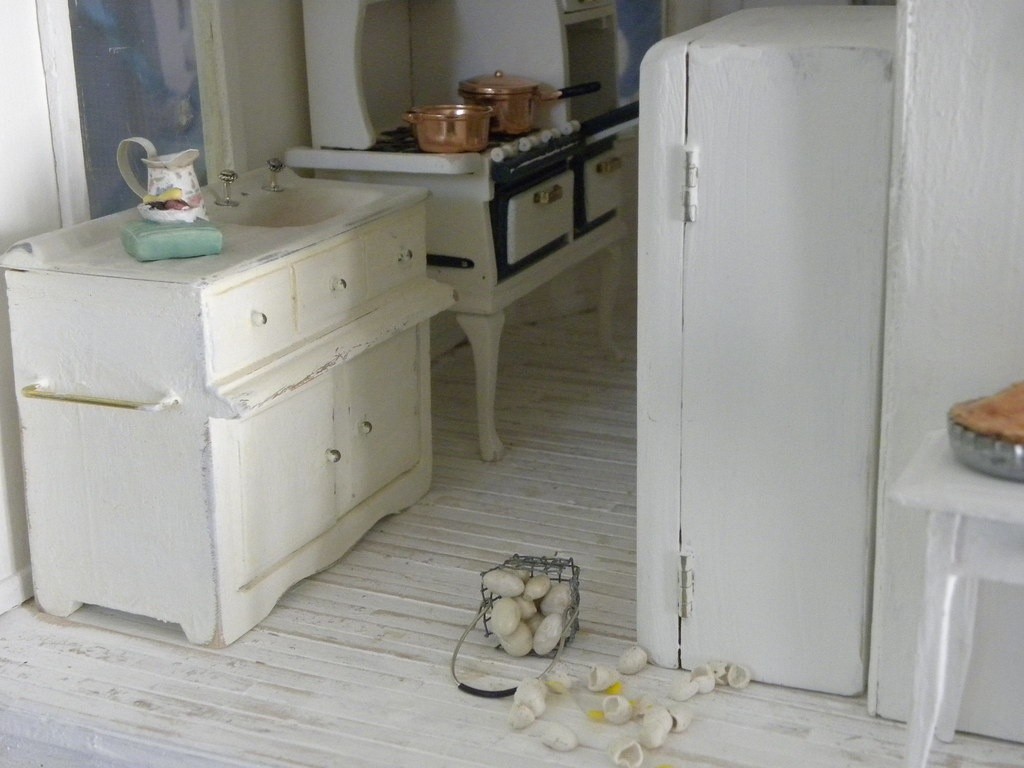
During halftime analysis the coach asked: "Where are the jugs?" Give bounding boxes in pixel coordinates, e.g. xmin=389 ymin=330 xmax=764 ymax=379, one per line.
xmin=116 ymin=132 xmax=208 ymax=218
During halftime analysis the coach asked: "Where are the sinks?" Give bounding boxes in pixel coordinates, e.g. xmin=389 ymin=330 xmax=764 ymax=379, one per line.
xmin=223 ymin=185 xmax=382 ymax=228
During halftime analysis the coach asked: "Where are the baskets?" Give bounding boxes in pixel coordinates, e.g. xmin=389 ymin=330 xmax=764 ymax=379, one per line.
xmin=480 ymin=552 xmax=580 ymax=659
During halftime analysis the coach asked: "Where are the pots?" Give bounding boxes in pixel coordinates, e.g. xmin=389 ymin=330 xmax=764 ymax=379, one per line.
xmin=401 ymin=100 xmax=496 ymax=152
xmin=456 ymin=68 xmax=603 ymax=137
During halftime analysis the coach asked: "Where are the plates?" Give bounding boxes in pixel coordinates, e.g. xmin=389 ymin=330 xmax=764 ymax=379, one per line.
xmin=947 ymin=396 xmax=1023 ymax=484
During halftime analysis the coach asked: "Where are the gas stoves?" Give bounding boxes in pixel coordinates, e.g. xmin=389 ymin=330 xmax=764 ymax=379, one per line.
xmin=374 ymin=113 xmax=583 ymax=182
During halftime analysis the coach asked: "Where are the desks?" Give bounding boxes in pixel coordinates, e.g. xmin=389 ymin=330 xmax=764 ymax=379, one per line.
xmin=883 ymin=423 xmax=1021 ymax=767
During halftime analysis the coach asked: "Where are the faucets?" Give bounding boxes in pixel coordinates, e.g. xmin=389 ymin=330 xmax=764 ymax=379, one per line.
xmin=260 ymin=158 xmax=287 ymax=192
xmin=215 ymin=169 xmax=241 ymax=208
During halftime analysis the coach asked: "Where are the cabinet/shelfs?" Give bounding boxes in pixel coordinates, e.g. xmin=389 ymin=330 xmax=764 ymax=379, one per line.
xmin=0 ymin=156 xmax=456 ymax=651
xmin=279 ymin=1 xmax=636 ymax=462
